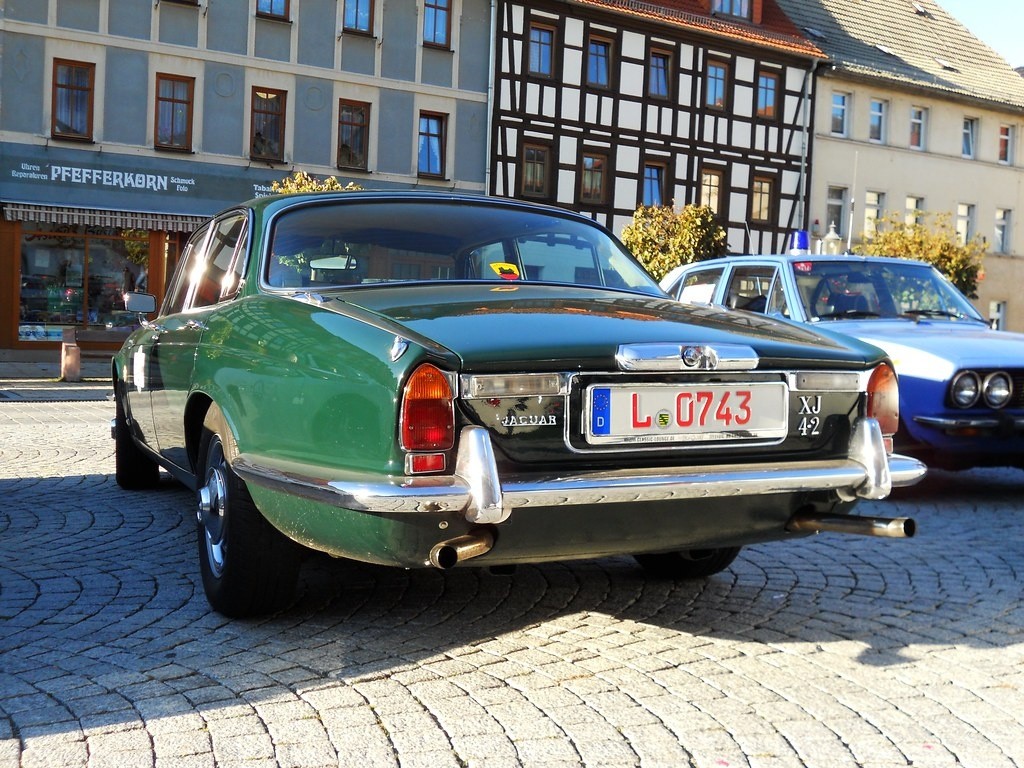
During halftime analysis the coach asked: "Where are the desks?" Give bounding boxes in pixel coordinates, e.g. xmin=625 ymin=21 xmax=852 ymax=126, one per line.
xmin=111 ymin=310 xmax=147 ymax=326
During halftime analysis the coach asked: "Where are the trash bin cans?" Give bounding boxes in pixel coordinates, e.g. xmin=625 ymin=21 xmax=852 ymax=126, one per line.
xmin=989 ymin=299 xmax=1004 ymax=330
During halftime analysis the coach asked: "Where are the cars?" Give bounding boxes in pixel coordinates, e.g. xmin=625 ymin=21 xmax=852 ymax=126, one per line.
xmin=656 ymin=232 xmax=1024 ymax=475
xmin=109 ymin=190 xmax=930 ymax=623
xmin=20 ymin=259 xmax=147 ymax=326
